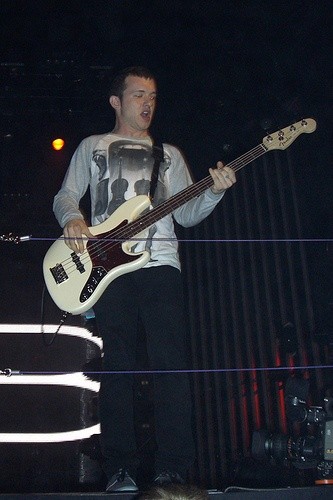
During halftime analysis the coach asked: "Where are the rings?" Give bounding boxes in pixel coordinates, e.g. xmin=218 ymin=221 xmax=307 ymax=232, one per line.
xmin=224 ymin=175 xmax=230 ymax=179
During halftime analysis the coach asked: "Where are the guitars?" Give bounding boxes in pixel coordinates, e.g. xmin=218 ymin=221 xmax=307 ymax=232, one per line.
xmin=43 ymin=117 xmax=317 ymax=315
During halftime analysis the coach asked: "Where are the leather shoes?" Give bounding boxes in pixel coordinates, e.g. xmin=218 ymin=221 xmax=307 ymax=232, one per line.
xmin=105 ymin=467 xmax=140 ymax=492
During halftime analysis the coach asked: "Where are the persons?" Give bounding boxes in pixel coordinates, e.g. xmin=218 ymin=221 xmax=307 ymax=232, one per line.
xmin=52 ymin=70 xmax=239 ymax=493
